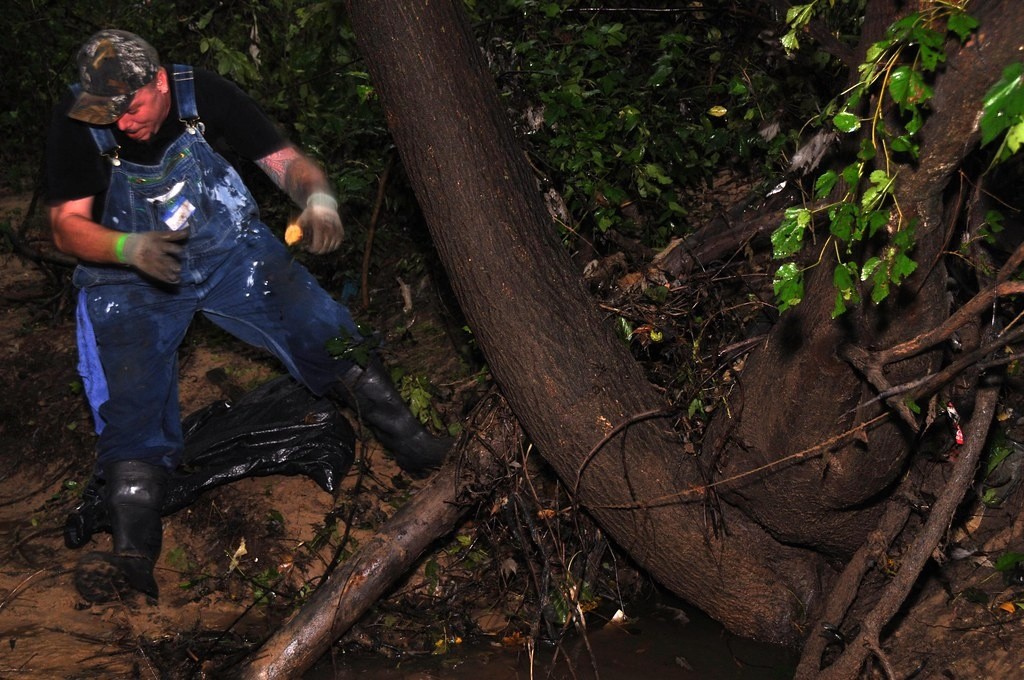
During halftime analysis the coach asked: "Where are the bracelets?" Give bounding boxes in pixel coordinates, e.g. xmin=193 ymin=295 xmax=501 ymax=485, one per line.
xmin=115 ymin=233 xmax=130 ymax=263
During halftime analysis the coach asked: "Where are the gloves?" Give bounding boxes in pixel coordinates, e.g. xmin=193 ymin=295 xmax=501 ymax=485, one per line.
xmin=123 ymin=232 xmax=188 ymax=284
xmin=296 ymin=193 xmax=344 ymax=254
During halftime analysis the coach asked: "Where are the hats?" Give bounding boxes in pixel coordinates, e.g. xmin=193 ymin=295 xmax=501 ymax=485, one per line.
xmin=68 ymin=30 xmax=159 ymax=124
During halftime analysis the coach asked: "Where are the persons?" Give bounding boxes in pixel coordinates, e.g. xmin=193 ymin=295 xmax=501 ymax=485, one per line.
xmin=42 ymin=29 xmax=456 ymax=604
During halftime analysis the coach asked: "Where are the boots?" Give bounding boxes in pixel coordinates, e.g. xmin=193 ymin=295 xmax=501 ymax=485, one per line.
xmin=337 ymin=354 xmax=455 ymax=481
xmin=73 ymin=460 xmax=163 ymax=608
